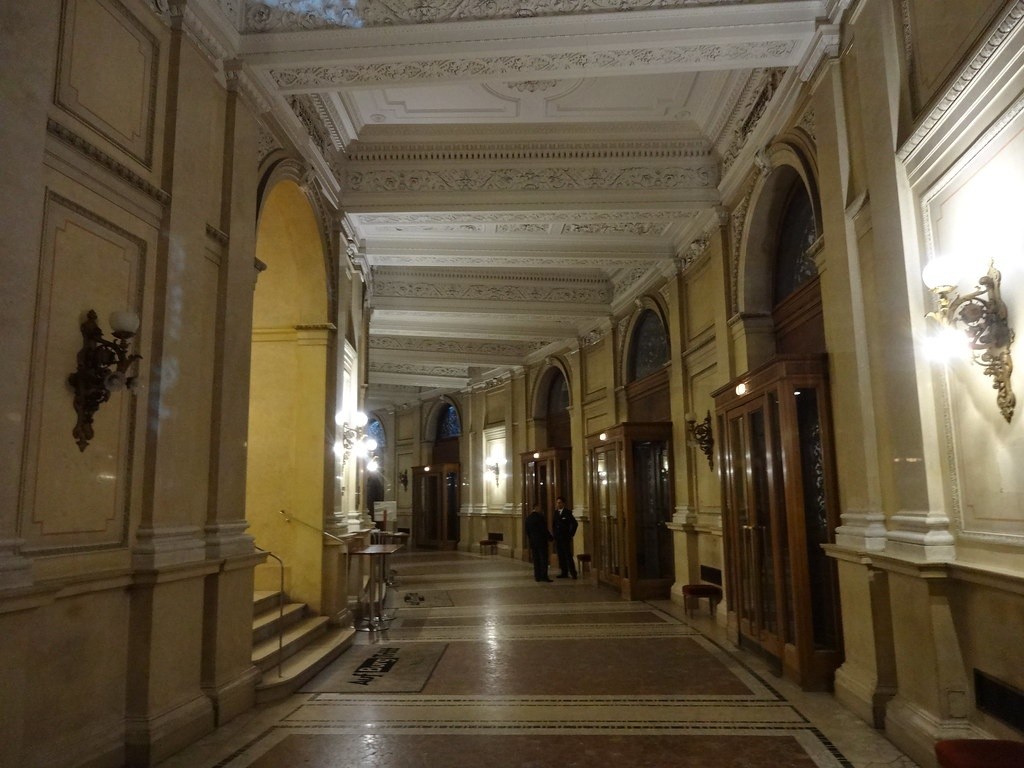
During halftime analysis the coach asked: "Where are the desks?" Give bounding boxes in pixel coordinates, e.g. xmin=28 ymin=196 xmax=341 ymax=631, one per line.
xmin=352 ymin=544 xmax=404 ymax=632
xmin=375 ymin=530 xmax=410 ymax=588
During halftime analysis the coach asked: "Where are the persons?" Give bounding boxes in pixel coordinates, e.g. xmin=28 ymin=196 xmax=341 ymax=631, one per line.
xmin=551 ymin=498 xmax=579 ymax=579
xmin=525 ymin=503 xmax=554 ymax=583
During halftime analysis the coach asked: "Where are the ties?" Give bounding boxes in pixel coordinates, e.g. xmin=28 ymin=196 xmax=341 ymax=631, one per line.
xmin=559 ymin=511 xmax=560 ymax=514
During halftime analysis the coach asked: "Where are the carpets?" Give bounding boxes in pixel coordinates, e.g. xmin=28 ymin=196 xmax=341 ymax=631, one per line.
xmin=296 ymin=641 xmax=450 ymax=693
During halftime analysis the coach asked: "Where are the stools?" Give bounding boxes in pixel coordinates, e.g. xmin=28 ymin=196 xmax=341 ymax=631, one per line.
xmin=933 ymin=738 xmax=1024 ymax=768
xmin=683 ymin=585 xmax=722 ymax=621
xmin=480 ymin=540 xmax=498 ymax=557
xmin=577 ymin=553 xmax=592 ymax=575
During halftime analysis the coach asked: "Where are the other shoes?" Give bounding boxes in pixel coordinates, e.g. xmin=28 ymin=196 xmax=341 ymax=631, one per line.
xmin=557 ymin=574 xmax=568 ymax=578
xmin=572 ymin=575 xmax=577 ymax=579
xmin=536 ymin=576 xmax=553 ymax=582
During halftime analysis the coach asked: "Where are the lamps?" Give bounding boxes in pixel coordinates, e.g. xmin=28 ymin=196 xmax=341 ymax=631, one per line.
xmin=397 ymin=466 xmax=408 ymax=492
xmin=343 ymin=411 xmax=377 ymax=467
xmin=685 ymin=410 xmax=714 ymax=473
xmin=486 ymin=457 xmax=500 ymax=488
xmin=920 ymin=251 xmax=1016 ymax=423
xmin=69 ymin=309 xmax=143 ymax=453
